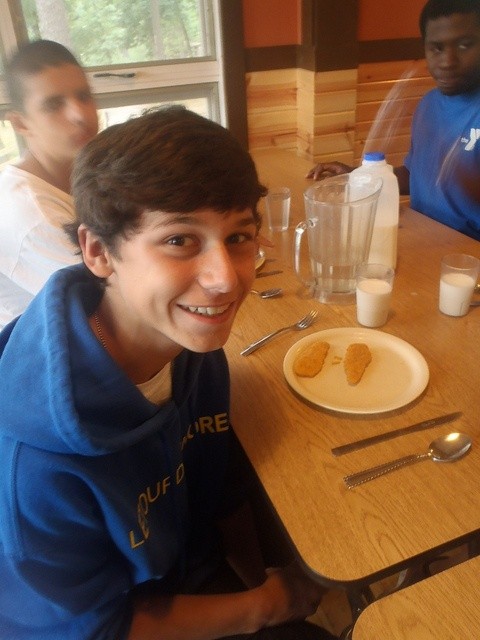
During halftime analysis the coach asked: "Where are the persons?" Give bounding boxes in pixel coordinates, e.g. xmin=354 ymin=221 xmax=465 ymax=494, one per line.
xmin=0 ymin=40 xmax=98 ymax=333
xmin=0 ymin=104 xmax=339 ymax=640
xmin=304 ymin=0 xmax=480 ymax=243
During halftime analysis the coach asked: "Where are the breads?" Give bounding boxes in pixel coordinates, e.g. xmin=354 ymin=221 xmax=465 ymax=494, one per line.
xmin=293 ymin=342 xmax=330 ymax=377
xmin=344 ymin=343 xmax=373 ymax=386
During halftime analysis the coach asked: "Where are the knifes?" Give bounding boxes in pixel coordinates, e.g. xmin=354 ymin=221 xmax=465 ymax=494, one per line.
xmin=331 ymin=411 xmax=463 ymax=458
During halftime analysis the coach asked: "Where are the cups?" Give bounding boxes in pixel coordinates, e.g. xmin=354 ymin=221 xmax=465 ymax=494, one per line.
xmin=355 ymin=264 xmax=395 ymax=328
xmin=291 ymin=172 xmax=383 ymax=304
xmin=438 ymin=253 xmax=480 ymax=317
xmin=265 ymin=187 xmax=291 ymax=232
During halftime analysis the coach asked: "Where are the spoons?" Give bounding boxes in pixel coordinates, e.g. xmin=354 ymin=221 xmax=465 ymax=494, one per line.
xmin=248 ymin=288 xmax=283 ymax=299
xmin=344 ymin=432 xmax=472 ymax=490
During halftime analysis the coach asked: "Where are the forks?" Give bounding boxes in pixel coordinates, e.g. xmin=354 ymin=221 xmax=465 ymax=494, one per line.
xmin=240 ymin=310 xmax=319 ymax=357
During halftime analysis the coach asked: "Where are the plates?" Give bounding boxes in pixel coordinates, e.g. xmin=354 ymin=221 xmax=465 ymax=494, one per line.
xmin=283 ymin=327 xmax=430 ymax=415
